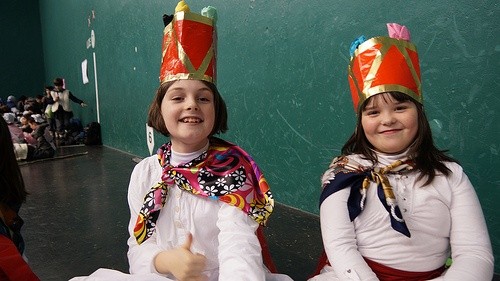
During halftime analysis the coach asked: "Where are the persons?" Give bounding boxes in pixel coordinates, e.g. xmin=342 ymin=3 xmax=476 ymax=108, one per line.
xmin=125 ymin=68 xmax=293 ymax=281
xmin=0 ymin=78 xmax=87 ymax=158
xmin=307 ymin=75 xmax=494 ymax=281
